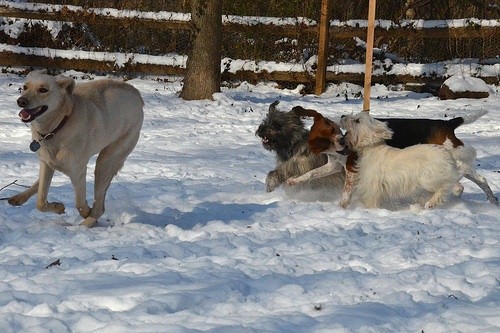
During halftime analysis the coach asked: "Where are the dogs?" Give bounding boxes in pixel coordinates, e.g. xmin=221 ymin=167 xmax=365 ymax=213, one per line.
xmin=255 ymin=100 xmax=499 ymax=211
xmin=8 ymin=69 xmax=145 ymax=228
